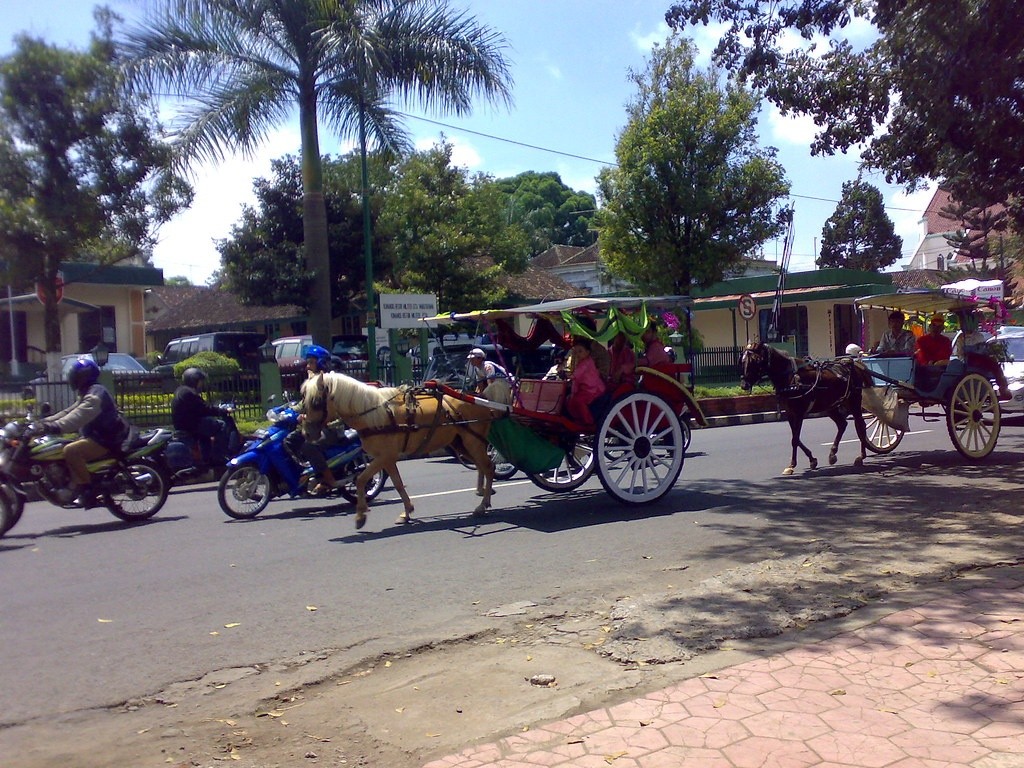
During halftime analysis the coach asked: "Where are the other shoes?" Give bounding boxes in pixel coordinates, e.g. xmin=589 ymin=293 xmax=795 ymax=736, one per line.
xmin=209 ymin=456 xmax=228 ymax=466
xmin=57 ymin=482 xmax=93 ymax=501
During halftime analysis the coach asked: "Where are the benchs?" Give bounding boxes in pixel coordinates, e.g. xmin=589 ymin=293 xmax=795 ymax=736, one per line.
xmin=941 ymin=332 xmax=1000 ymax=342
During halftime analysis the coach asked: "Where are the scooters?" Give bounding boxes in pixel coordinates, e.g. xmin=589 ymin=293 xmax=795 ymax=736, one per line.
xmin=132 ymin=401 xmax=255 ymax=495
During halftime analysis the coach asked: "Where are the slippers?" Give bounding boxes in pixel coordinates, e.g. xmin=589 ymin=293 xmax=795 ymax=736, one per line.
xmin=314 ymin=481 xmax=336 ymax=491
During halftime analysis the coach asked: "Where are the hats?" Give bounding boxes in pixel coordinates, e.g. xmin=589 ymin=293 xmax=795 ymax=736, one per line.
xmin=932 ymin=314 xmax=945 ymax=325
xmin=467 ymin=348 xmax=486 ymax=358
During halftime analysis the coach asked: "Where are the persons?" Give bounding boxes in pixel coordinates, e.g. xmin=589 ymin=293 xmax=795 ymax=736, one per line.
xmin=915 ymin=314 xmax=952 ymax=367
xmin=170 ymin=366 xmax=232 ymax=472
xmin=284 ymin=344 xmax=345 ymax=495
xmin=876 ymin=311 xmax=915 ymax=357
xmin=467 ymin=349 xmax=519 ymax=391
xmin=542 ymin=317 xmax=673 ymax=426
xmin=952 ymin=319 xmax=985 ymax=362
xmin=37 ymin=358 xmax=131 ymax=507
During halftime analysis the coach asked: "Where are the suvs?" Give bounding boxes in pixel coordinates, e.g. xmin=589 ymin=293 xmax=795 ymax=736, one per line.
xmin=151 ymin=331 xmax=278 ymax=374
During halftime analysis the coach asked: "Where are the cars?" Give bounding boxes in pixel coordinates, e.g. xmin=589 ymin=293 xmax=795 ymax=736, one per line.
xmin=254 ymin=334 xmax=553 ymax=390
xmin=24 ymin=353 xmax=161 ymax=400
xmin=949 ymin=326 xmax=1024 ymax=414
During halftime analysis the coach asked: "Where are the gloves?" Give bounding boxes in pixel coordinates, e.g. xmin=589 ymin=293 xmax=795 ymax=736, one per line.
xmin=37 ymin=418 xmax=61 ymax=437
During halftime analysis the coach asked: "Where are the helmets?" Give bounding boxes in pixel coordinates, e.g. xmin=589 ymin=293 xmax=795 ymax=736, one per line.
xmin=300 ymin=344 xmax=331 ymax=369
xmin=182 ymin=368 xmax=207 ymax=393
xmin=68 ymin=359 xmax=100 ymax=385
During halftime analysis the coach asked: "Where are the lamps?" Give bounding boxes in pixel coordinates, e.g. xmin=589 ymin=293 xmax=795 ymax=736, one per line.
xmin=89 ymin=341 xmax=110 ymax=370
xmin=668 ymin=331 xmax=684 ymax=346
xmin=258 ymin=338 xmax=278 ymax=361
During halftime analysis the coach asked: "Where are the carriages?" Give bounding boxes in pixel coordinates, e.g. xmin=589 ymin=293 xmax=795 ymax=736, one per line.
xmin=301 ymin=295 xmax=709 ymax=529
xmin=739 ymin=290 xmax=1012 ymax=475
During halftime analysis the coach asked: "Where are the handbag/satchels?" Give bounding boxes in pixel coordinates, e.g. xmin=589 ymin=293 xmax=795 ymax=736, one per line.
xmin=104 ymin=414 xmax=139 ymax=455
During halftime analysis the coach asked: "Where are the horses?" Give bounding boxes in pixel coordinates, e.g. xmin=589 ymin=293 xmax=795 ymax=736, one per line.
xmin=740 ymin=338 xmax=875 ymax=475
xmin=300 ymin=370 xmax=511 ymax=529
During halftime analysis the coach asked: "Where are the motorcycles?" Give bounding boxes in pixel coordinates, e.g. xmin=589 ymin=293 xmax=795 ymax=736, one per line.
xmin=217 ymin=394 xmax=390 ymax=520
xmin=0 ymin=403 xmax=171 ymax=538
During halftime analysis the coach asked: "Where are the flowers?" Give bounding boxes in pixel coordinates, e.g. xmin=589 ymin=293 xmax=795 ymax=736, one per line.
xmin=963 ymin=293 xmax=1018 ymax=337
